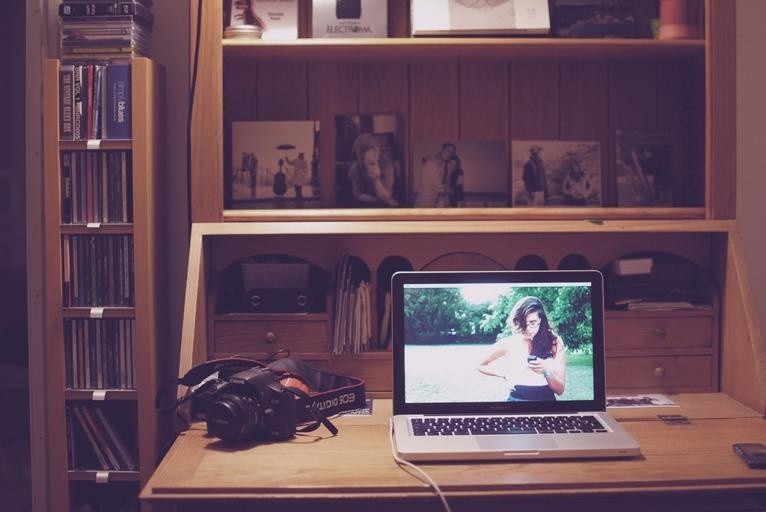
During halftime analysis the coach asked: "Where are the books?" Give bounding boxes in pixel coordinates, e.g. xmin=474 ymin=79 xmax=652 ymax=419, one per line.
xmin=58 ymin=16 xmax=143 ymax=475
xmin=331 ymin=255 xmax=371 ymax=359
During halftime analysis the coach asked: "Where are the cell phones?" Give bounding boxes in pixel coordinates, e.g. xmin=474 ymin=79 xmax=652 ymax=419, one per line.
xmin=527 ymin=355 xmax=537 ymax=364
xmin=733 ymin=443 xmax=766 ymax=469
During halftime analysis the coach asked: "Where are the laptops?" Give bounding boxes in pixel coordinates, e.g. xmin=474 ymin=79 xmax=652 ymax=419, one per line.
xmin=390 ymin=271 xmax=641 ymax=463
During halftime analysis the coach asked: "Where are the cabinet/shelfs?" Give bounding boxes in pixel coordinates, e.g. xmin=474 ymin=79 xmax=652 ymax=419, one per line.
xmin=40 ymin=57 xmax=166 ymax=511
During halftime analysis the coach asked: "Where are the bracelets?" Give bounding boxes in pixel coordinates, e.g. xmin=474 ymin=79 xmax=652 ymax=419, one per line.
xmin=542 ymin=370 xmax=552 ymax=378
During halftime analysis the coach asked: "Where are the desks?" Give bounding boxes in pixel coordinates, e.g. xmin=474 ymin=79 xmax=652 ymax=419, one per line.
xmin=140 ymin=0 xmax=766 ymax=511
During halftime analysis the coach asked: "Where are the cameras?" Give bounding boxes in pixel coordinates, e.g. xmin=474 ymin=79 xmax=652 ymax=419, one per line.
xmin=207 ymin=367 xmax=296 ymax=442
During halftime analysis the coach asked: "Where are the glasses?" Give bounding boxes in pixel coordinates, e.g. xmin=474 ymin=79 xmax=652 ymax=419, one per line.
xmin=526 ymin=317 xmax=542 ymax=328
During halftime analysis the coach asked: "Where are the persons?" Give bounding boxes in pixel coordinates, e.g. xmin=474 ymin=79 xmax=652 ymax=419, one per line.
xmin=564 ymin=161 xmax=596 ymax=203
xmin=350 ymin=132 xmax=402 ymax=208
xmin=274 ymin=160 xmax=292 ymax=193
xmin=523 ymin=146 xmax=547 ymax=204
xmin=477 ymin=296 xmax=569 ymax=401
xmin=439 ymin=156 xmax=463 ymax=210
xmin=414 ymin=144 xmax=459 ymax=208
xmin=286 ymin=153 xmax=310 ymax=195
